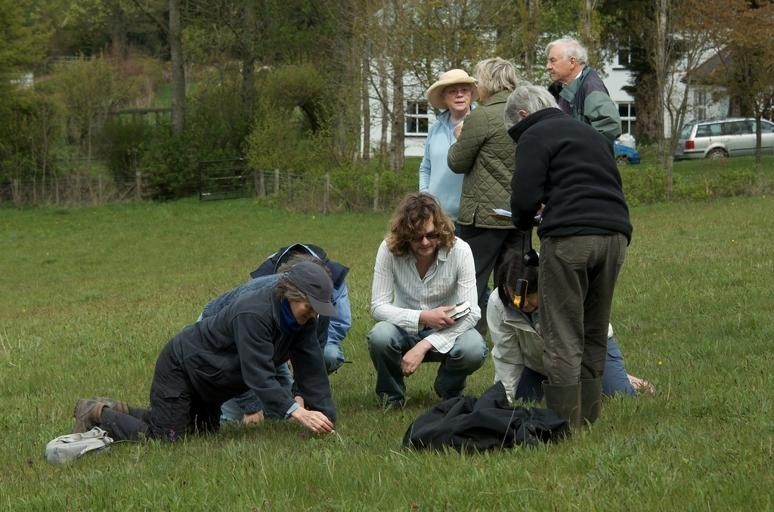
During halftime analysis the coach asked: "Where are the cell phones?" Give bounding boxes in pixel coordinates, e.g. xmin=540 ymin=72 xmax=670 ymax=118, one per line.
xmin=514 ymin=279 xmax=528 ymax=309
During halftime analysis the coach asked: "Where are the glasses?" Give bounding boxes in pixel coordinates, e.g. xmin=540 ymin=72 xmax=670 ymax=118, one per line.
xmin=406 ymin=232 xmax=440 ymax=242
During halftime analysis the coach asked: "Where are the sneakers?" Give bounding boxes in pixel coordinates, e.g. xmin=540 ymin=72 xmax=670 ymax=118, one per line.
xmin=626 ymin=372 xmax=657 ymax=397
xmin=376 ymin=380 xmax=464 ymax=409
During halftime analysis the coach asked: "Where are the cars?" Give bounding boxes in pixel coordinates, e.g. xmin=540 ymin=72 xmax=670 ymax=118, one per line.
xmin=613 ymin=143 xmax=640 ymax=168
xmin=674 ymin=117 xmax=774 ymax=160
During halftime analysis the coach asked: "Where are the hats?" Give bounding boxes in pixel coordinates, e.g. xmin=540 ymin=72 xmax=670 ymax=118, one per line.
xmin=285 ymin=262 xmax=339 ymax=318
xmin=425 ymin=68 xmax=481 ymax=111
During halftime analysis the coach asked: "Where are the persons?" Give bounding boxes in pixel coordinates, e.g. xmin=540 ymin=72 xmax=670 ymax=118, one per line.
xmin=444 ymin=57 xmax=534 ymax=330
xmin=542 ymin=34 xmax=623 ymax=144
xmin=194 ymin=253 xmax=334 ymax=426
xmin=70 ymin=263 xmax=337 ymax=439
xmin=248 ymin=242 xmax=353 ymax=376
xmin=366 ymin=189 xmax=489 ymax=408
xmin=416 ymin=67 xmax=477 ymax=236
xmin=503 ymin=84 xmax=633 ymax=435
xmin=471 ymin=252 xmax=655 ymax=413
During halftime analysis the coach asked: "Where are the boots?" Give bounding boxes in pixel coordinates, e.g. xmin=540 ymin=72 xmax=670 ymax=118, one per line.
xmin=540 ymin=380 xmax=581 ymax=440
xmin=92 ymin=395 xmax=129 ymax=416
xmin=71 ymin=398 xmax=108 ymax=432
xmin=580 ymin=377 xmax=603 ymax=426
